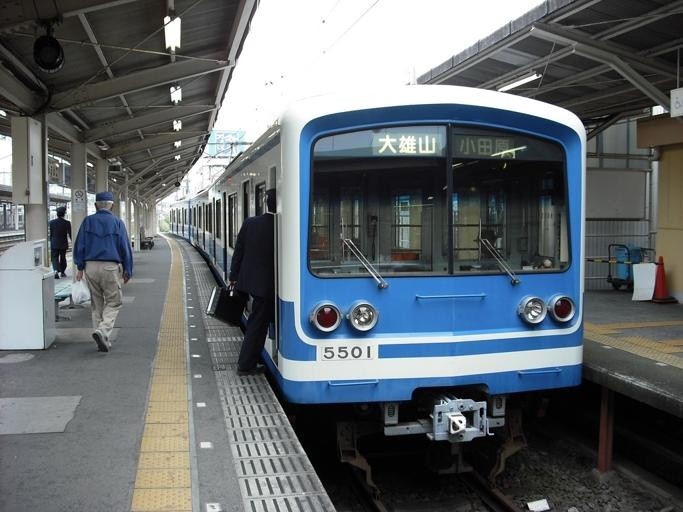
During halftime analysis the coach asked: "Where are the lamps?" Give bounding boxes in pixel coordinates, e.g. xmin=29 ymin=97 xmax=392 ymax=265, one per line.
xmin=33 ymin=36 xmax=65 ymax=75
xmin=495 ymin=70 xmax=543 ymax=92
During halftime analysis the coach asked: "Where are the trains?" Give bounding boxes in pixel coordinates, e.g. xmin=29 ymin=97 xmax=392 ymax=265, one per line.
xmin=170 ymin=85 xmax=586 ymax=498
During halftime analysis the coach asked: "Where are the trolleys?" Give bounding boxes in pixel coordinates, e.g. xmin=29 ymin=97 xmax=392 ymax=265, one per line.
xmin=606 ymin=242 xmax=648 ymax=293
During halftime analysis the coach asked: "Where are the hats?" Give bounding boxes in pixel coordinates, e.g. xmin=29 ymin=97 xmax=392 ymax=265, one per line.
xmin=56 ymin=205 xmax=67 ymax=212
xmin=264 ymin=187 xmax=277 ymax=196
xmin=94 ymin=192 xmax=114 ymax=202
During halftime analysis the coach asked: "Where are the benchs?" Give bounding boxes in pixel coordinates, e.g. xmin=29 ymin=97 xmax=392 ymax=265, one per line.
xmin=140 ymin=232 xmax=153 ymax=249
xmin=53 ymin=276 xmax=75 ymax=322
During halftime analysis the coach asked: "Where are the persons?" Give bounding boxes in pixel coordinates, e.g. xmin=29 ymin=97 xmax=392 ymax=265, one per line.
xmin=73 ymin=192 xmax=134 ymax=352
xmin=228 ymin=188 xmax=275 ymax=376
xmin=48 ymin=206 xmax=71 ymax=280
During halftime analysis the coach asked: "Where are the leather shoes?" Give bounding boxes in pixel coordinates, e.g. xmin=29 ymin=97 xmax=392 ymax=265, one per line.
xmin=54 ymin=270 xmax=68 ymax=279
xmin=235 ymin=364 xmax=267 ymax=375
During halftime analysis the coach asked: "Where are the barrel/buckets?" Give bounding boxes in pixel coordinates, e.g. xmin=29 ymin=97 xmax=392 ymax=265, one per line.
xmin=615 ymin=245 xmax=641 ymax=278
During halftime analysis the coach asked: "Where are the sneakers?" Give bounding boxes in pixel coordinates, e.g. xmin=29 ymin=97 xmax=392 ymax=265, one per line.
xmin=91 ymin=329 xmax=110 ymax=351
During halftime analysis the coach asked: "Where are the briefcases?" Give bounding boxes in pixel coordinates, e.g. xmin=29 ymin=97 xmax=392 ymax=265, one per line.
xmin=205 ymin=284 xmax=244 ymax=328
